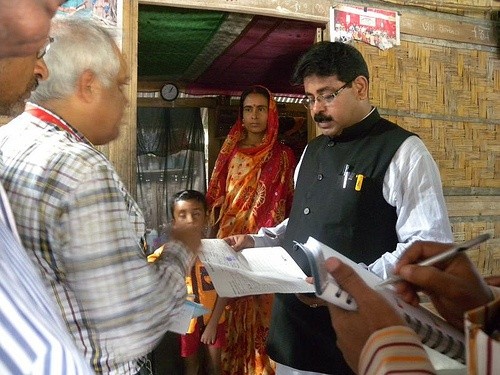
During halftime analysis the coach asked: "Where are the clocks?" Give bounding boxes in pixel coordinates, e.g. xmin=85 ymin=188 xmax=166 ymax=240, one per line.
xmin=160 ymin=83 xmax=179 ymax=102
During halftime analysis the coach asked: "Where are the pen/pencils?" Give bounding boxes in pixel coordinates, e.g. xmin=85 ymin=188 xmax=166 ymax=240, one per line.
xmin=342 ymin=164 xmax=350 ymax=188
xmin=374 ymin=233 xmax=491 ymax=286
xmin=355 ymin=172 xmax=364 ymax=191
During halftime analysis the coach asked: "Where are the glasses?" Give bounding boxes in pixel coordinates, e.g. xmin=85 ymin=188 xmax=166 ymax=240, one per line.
xmin=302 ymin=72 xmax=369 ymax=109
xmin=0 ymin=36 xmax=54 ymax=60
